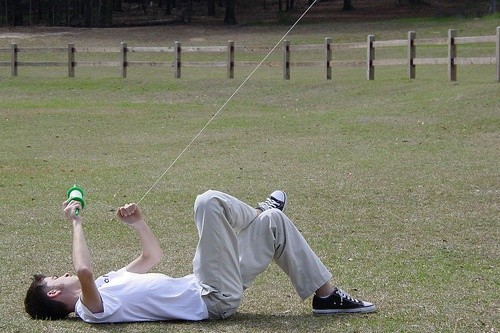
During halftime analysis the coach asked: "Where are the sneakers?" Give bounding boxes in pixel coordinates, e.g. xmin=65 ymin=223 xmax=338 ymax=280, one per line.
xmin=256 ymin=189 xmax=287 ymax=216
xmin=312 ymin=286 xmax=377 ymax=316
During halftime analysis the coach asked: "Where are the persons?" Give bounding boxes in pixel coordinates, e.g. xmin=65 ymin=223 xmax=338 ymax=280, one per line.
xmin=24 ymin=189 xmax=377 ymax=323
